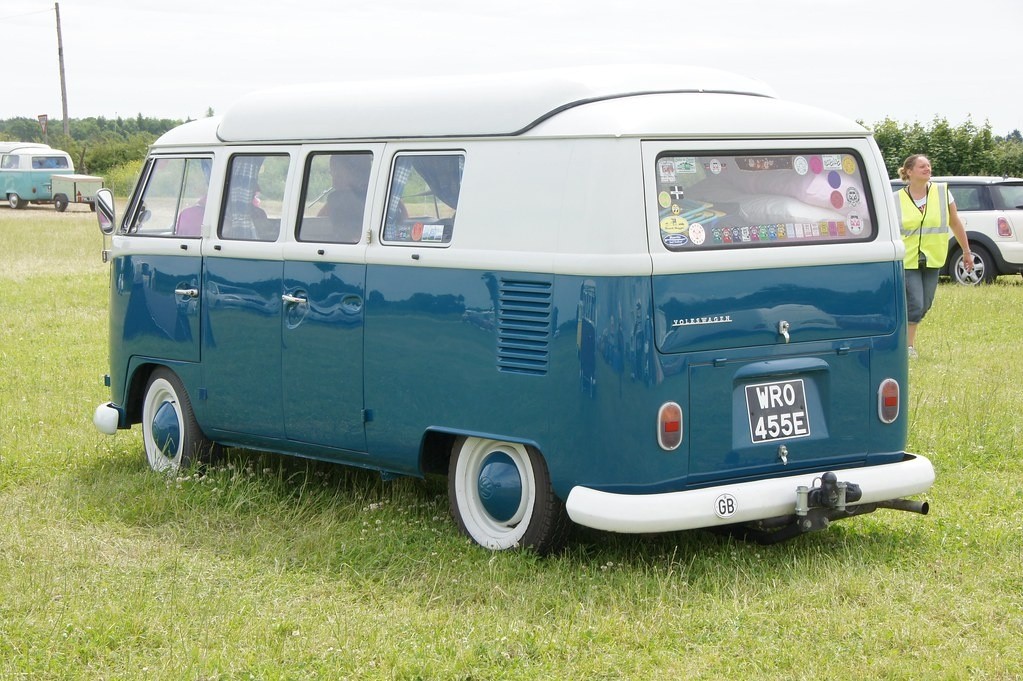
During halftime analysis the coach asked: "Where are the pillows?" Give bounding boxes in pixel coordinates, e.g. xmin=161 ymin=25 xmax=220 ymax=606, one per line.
xmin=738 ymin=195 xmax=847 ymax=226
xmin=723 ymin=163 xmax=869 ymax=219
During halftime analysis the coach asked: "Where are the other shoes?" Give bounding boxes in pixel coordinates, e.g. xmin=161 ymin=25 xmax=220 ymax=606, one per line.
xmin=907 ymin=346 xmax=917 ymax=359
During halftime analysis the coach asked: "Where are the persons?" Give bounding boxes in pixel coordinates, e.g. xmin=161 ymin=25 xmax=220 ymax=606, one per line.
xmin=316 ymin=154 xmax=410 ymax=219
xmin=894 ymin=154 xmax=974 ymax=359
xmin=176 ymin=159 xmax=269 ymax=238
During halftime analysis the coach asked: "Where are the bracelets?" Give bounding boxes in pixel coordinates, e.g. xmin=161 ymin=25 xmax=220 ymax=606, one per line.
xmin=963 ymin=250 xmax=971 ymax=254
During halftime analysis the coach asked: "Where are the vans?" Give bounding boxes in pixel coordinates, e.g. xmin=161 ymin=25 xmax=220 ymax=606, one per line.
xmin=93 ymin=86 xmax=939 ymax=561
xmin=886 ymin=175 xmax=1023 ymax=288
xmin=0 ymin=142 xmax=75 ymax=209
